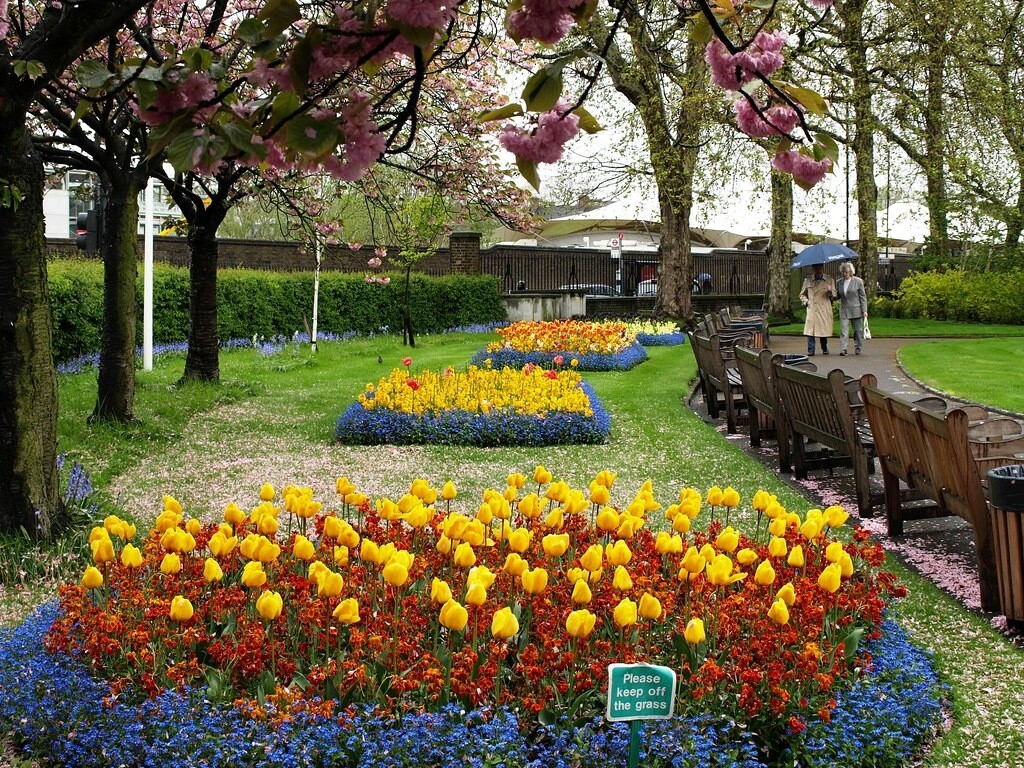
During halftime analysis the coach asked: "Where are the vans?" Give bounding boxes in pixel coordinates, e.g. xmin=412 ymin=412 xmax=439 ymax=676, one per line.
xmin=634 ymin=277 xmax=703 ymax=296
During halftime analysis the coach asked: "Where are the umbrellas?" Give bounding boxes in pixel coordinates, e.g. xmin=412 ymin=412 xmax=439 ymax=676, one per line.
xmin=790 ymin=242 xmax=860 ymax=277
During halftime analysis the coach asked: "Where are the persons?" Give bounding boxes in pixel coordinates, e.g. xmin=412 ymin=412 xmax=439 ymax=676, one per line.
xmin=877 ymin=282 xmax=883 ymax=297
xmin=516 ymin=281 xmax=528 ymax=290
xmin=834 ymin=262 xmax=868 ymax=357
xmin=799 ymin=264 xmax=837 ymax=357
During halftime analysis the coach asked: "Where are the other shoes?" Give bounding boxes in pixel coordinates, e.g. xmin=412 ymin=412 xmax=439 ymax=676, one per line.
xmin=840 ymin=350 xmax=847 ymax=356
xmin=807 ymin=352 xmax=814 ymax=356
xmin=855 ymin=348 xmax=861 ymax=355
xmin=823 ymin=350 xmax=829 ymax=354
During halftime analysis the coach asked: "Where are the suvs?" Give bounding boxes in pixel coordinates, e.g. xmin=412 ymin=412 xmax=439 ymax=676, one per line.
xmin=558 ymin=284 xmax=622 ymax=297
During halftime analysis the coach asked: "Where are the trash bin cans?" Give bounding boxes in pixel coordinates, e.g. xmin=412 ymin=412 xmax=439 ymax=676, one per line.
xmin=986 ymin=464 xmax=1024 ymax=625
xmin=730 ymin=323 xmax=764 ymax=349
xmin=758 ymin=354 xmax=809 ymax=431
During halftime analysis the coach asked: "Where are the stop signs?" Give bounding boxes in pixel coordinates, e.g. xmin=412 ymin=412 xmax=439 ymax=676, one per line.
xmin=611 ymin=238 xmax=620 ymax=248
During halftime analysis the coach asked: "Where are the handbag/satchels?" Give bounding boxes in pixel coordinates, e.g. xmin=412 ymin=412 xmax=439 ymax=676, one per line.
xmin=863 ymin=317 xmax=871 ymax=339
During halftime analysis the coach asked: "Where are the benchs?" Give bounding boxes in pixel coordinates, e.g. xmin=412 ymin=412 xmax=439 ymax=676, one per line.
xmin=683 ymin=305 xmax=1024 ymax=611
